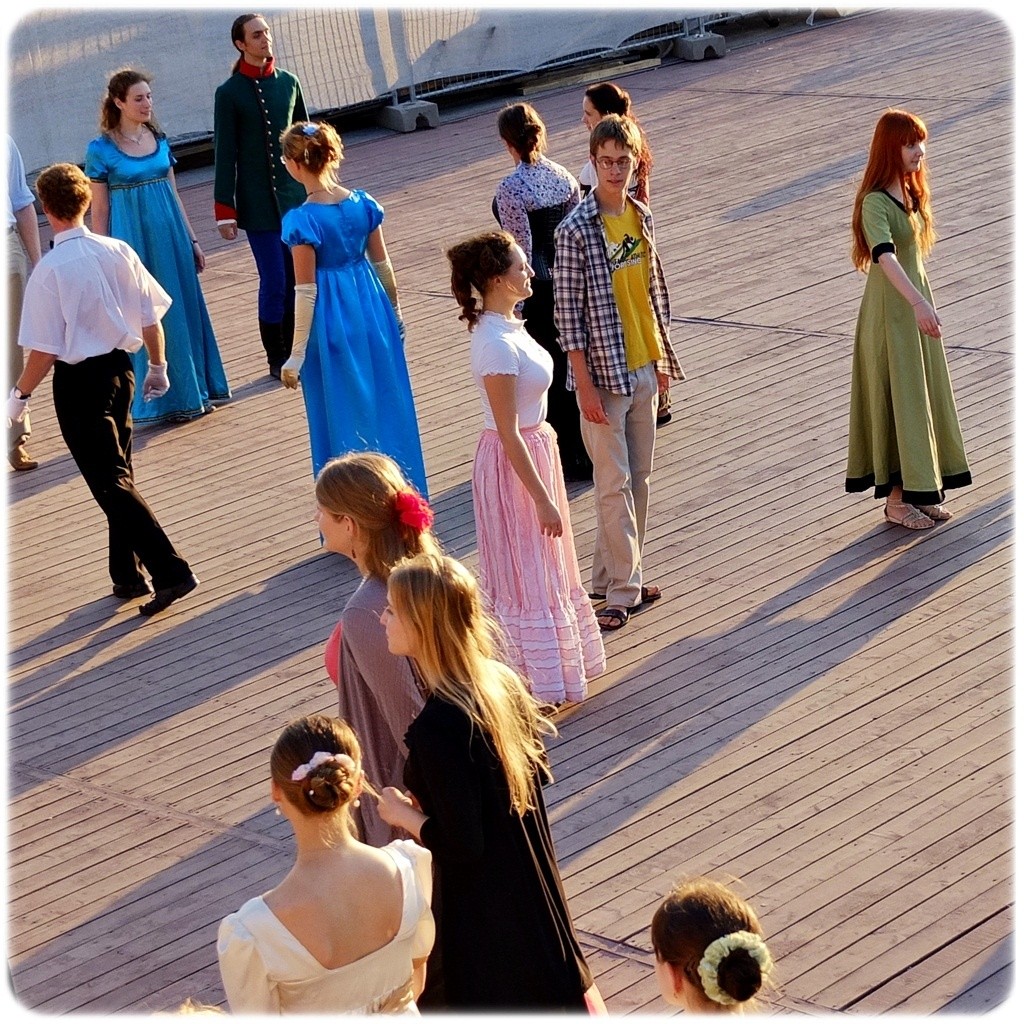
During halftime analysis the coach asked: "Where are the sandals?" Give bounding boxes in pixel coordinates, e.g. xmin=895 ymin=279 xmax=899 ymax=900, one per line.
xmin=883 ymin=497 xmax=936 ymax=529
xmin=914 ymin=504 xmax=953 ymax=520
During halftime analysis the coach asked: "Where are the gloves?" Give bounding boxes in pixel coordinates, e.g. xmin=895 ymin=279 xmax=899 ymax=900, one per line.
xmin=371 ymin=259 xmax=407 ymax=345
xmin=7 ymin=387 xmax=30 ymax=428
xmin=143 ymin=360 xmax=170 ymax=403
xmin=280 ymin=283 xmax=318 ymax=390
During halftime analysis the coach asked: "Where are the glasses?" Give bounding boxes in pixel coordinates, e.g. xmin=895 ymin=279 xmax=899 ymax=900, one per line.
xmin=594 ymin=157 xmax=633 ymax=170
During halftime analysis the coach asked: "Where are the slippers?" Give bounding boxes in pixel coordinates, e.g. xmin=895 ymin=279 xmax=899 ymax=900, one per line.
xmin=587 ymin=584 xmax=661 ymax=601
xmin=598 ymin=601 xmax=642 ymax=630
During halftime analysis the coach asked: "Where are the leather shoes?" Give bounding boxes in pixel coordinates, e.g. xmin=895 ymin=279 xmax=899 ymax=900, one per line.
xmin=113 ymin=580 xmax=151 ymax=599
xmin=138 ymin=573 xmax=200 ymax=616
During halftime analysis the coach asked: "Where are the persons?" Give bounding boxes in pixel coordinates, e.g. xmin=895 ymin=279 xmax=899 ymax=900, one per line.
xmin=85 ymin=71 xmax=232 ymax=428
xmin=213 ymin=13 xmax=311 ymax=381
xmin=492 ymin=103 xmax=594 ymax=482
xmin=447 ymin=229 xmax=608 ymax=703
xmin=380 ymin=552 xmax=594 ymax=1016
xmin=578 ymin=82 xmax=672 ymax=425
xmin=651 ymin=883 xmax=773 ymax=1015
xmin=217 ymin=714 xmax=435 ymax=1017
xmin=8 ymin=133 xmax=42 ymax=470
xmin=7 ymin=163 xmax=200 ymax=616
xmin=554 ymin=112 xmax=686 ymax=630
xmin=281 ymin=122 xmax=430 ymax=545
xmin=314 ymin=452 xmax=435 ymax=847
xmin=845 ymin=109 xmax=973 ymax=529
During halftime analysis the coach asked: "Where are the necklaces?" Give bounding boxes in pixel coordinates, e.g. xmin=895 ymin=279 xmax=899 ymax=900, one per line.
xmin=124 ymin=135 xmax=142 ymax=145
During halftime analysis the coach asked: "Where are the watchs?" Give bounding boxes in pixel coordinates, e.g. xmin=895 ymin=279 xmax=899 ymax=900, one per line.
xmin=14 ymin=387 xmax=31 ymax=400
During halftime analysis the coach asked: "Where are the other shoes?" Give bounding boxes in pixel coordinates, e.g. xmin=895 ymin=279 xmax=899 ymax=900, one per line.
xmin=10 ymin=446 xmax=38 ymax=469
xmin=167 ymin=417 xmax=193 ymax=421
xmin=656 ymin=413 xmax=672 ymax=424
xmin=205 ymin=405 xmax=215 ymax=413
xmin=270 ymin=367 xmax=301 ymax=382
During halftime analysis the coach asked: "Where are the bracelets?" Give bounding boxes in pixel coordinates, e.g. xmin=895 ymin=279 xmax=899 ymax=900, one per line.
xmin=192 ymin=240 xmax=198 ymax=243
